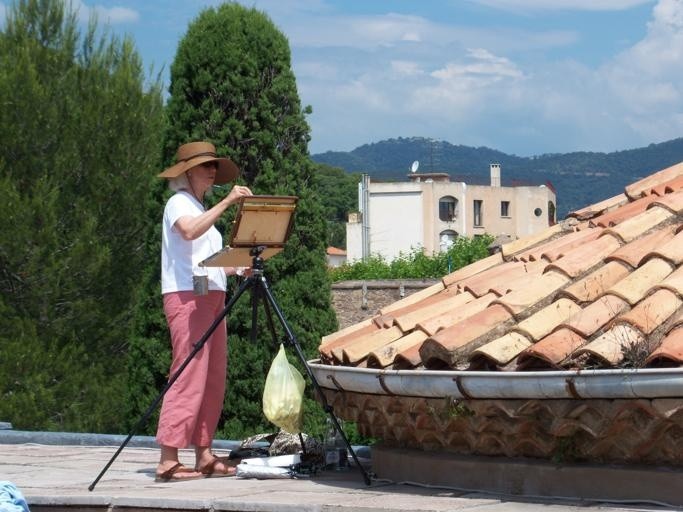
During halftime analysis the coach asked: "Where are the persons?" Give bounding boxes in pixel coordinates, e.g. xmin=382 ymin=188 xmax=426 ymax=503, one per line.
xmin=155 ymin=139 xmax=254 ymax=482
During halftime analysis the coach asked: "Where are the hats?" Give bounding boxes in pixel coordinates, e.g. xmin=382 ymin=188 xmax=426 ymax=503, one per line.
xmin=157 ymin=141 xmax=240 ymax=187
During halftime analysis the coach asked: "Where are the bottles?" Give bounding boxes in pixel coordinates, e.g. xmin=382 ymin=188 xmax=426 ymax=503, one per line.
xmin=324 ymin=418 xmax=349 ymax=471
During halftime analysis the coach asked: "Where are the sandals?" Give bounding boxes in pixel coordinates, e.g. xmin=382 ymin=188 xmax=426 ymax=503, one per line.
xmin=202 ymin=454 xmax=239 ymax=479
xmin=156 ymin=461 xmax=200 ymax=482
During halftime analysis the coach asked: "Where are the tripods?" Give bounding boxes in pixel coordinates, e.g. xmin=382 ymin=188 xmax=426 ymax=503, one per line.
xmin=88 ymin=256 xmax=371 ymax=492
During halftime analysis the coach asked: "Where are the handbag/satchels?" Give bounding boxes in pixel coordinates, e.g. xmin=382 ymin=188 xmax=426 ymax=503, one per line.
xmin=239 ymin=430 xmax=325 ymax=472
